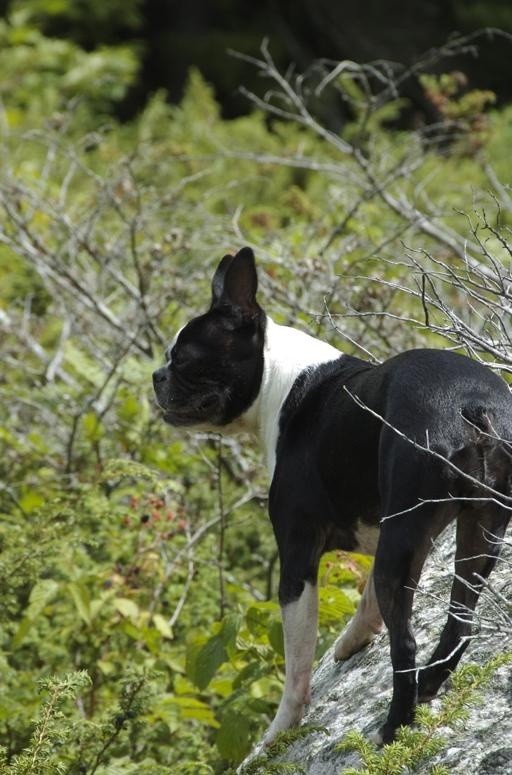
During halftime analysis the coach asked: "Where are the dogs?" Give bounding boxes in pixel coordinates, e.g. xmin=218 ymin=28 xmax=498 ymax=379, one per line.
xmin=151 ymin=247 xmax=512 ymax=775
